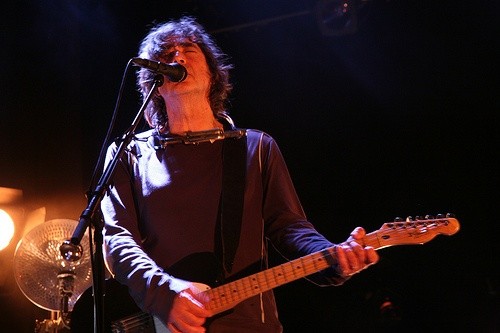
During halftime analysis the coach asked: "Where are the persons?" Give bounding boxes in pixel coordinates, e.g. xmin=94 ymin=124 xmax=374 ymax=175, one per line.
xmin=98 ymin=15 xmax=380 ymax=333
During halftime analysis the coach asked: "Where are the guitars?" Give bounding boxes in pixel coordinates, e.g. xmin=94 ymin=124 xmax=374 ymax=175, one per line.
xmin=70 ymin=212 xmax=462 ymax=333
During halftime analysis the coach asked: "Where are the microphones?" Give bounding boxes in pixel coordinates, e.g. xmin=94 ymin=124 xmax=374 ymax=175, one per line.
xmin=132 ymin=57 xmax=187 ymax=83
